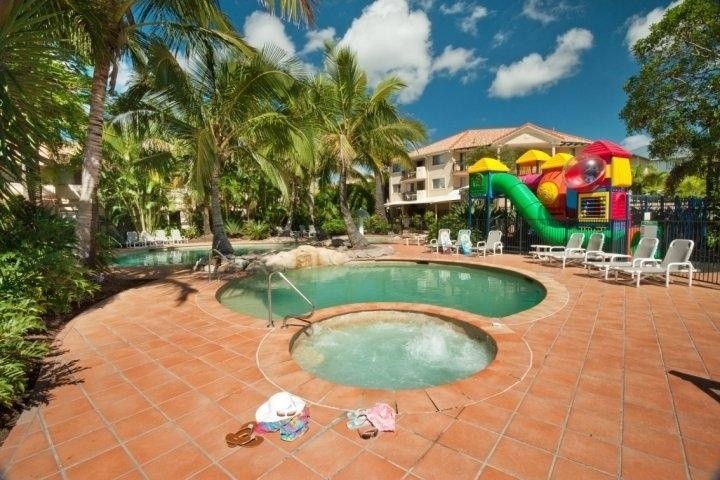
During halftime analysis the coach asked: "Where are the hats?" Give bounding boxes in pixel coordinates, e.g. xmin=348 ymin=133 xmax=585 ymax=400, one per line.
xmin=255 ymin=392 xmax=305 ymax=422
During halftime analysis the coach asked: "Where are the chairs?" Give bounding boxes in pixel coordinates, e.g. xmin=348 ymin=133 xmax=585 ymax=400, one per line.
xmin=276 ymin=225 xmax=318 ymax=238
xmin=529 ymin=233 xmax=702 ymax=286
xmin=424 ymin=228 xmax=504 ymax=258
xmin=126 ymin=229 xmax=188 ymax=249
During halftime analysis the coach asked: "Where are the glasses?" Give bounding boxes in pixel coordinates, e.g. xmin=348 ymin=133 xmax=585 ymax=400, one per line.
xmin=357 ymin=419 xmax=379 ymax=440
xmin=277 ymin=408 xmax=297 ymax=417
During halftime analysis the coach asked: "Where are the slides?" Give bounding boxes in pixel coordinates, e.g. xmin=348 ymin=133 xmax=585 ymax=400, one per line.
xmin=492 ymin=172 xmax=663 ymax=259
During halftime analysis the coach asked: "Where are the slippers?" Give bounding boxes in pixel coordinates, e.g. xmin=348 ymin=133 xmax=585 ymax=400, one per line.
xmin=346 ymin=408 xmax=368 ymax=430
xmin=225 ymin=421 xmax=264 ymax=448
